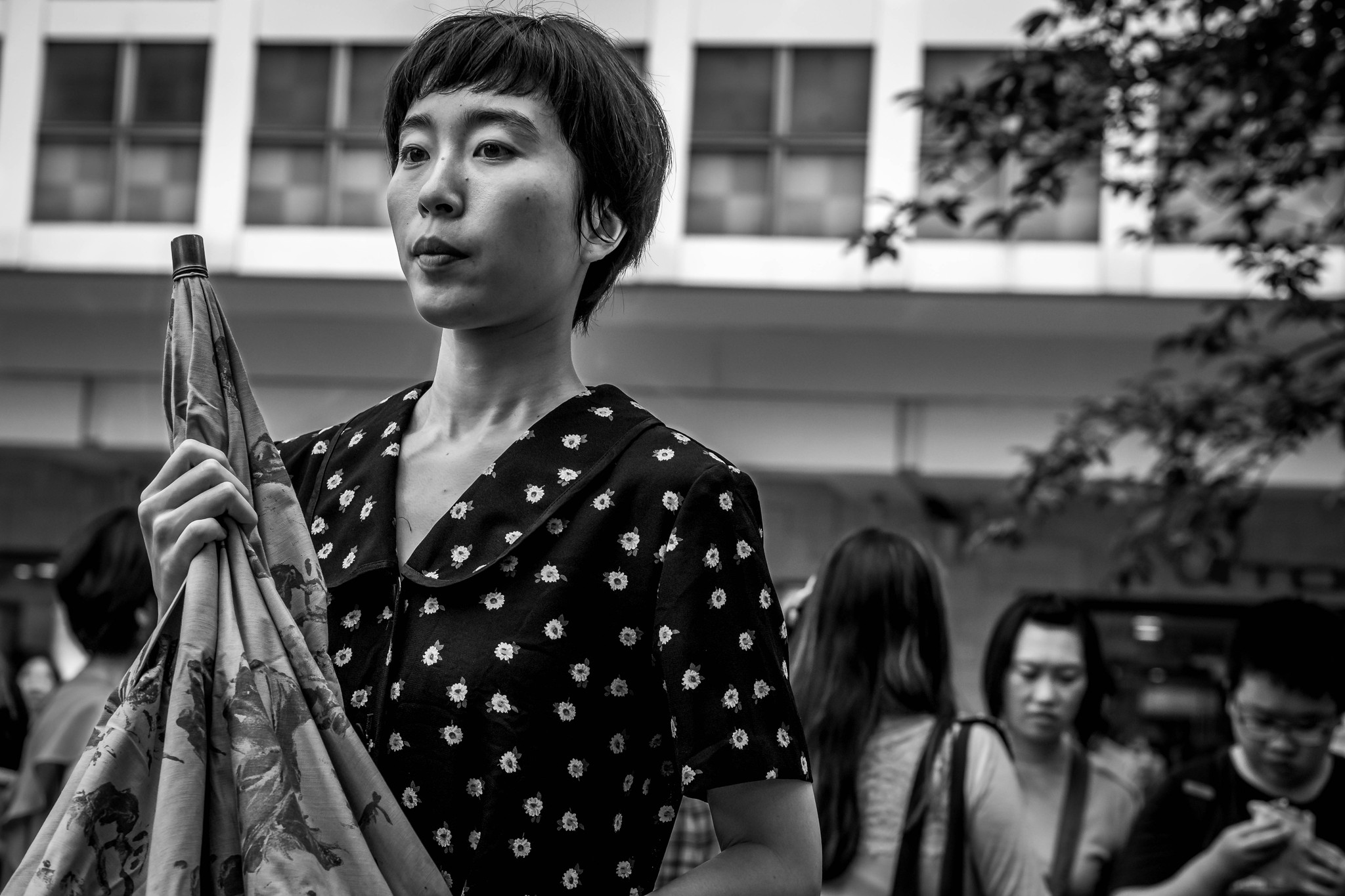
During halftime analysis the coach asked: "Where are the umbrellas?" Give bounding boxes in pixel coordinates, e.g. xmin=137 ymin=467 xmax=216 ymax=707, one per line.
xmin=1 ymin=232 xmax=469 ymax=894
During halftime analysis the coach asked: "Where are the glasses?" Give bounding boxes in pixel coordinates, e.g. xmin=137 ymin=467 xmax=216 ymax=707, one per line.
xmin=1229 ymin=691 xmax=1341 ymax=748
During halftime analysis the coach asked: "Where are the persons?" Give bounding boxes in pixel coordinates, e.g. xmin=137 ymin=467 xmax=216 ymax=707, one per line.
xmin=783 ymin=527 xmax=1055 ymax=896
xmin=1091 ymin=590 xmax=1344 ymax=896
xmin=1 ymin=504 xmax=168 ymax=879
xmin=96 ymin=8 xmax=822 ymax=895
xmin=964 ymin=584 xmax=1173 ymax=896
